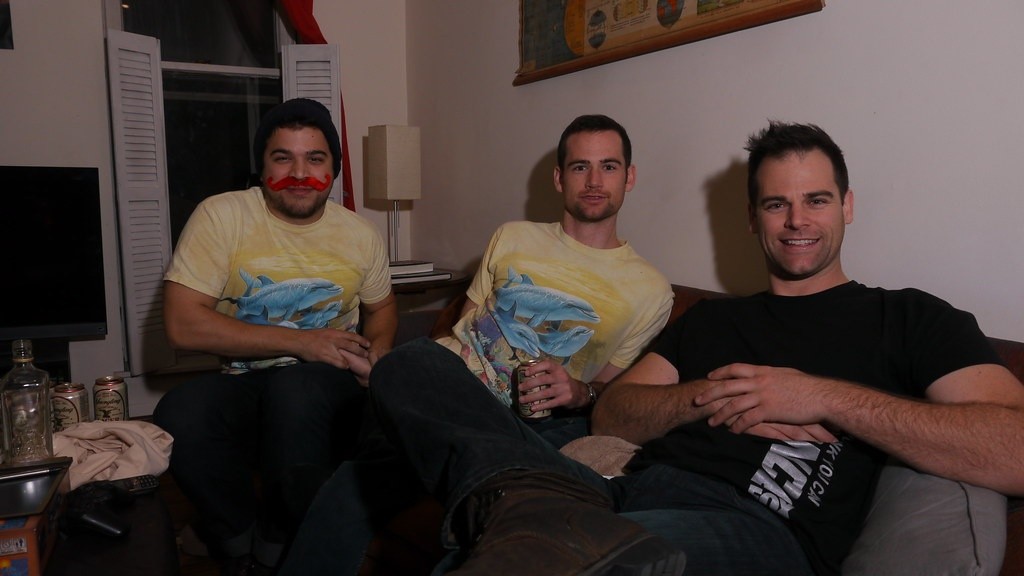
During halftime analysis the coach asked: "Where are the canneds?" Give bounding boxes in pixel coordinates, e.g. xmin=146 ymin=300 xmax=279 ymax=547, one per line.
xmin=92 ymin=375 xmax=129 ymax=421
xmin=51 ymin=383 xmax=90 ymax=432
xmin=517 ymin=359 xmax=552 ymax=420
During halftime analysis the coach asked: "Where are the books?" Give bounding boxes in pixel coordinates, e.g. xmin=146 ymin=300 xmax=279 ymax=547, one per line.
xmin=389 ymin=259 xmax=452 ymax=285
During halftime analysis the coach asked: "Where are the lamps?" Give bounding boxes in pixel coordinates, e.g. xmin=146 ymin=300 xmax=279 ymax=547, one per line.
xmin=366 ymin=123 xmax=422 ymax=261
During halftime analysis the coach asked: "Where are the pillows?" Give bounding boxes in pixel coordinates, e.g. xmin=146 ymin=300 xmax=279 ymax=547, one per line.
xmin=844 ymin=466 xmax=1009 ymax=576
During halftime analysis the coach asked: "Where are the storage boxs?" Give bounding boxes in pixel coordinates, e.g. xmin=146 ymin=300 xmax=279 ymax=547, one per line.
xmin=0 ymin=470 xmax=72 ymax=576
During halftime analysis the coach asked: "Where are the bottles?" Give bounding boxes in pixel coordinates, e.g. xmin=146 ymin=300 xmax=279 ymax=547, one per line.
xmin=0 ymin=340 xmax=54 ymax=464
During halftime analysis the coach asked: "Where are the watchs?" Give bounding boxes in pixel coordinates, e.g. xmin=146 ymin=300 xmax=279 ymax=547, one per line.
xmin=575 ymin=383 xmax=597 ymax=413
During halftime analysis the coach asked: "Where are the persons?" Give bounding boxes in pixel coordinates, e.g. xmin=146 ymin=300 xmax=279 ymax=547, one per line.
xmin=152 ymin=98 xmax=399 ymax=576
xmin=369 ymin=124 xmax=1023 ymax=576
xmin=281 ymin=114 xmax=673 ymax=576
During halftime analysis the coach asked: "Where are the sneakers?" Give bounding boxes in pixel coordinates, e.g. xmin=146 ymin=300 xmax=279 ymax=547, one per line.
xmin=441 ymin=469 xmax=687 ymax=576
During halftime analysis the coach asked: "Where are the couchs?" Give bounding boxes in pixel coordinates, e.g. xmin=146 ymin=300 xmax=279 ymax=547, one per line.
xmin=433 ymin=285 xmax=1024 ymax=576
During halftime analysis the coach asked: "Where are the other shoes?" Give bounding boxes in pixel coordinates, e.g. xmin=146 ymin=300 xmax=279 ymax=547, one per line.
xmin=220 ymin=552 xmax=277 ymax=576
